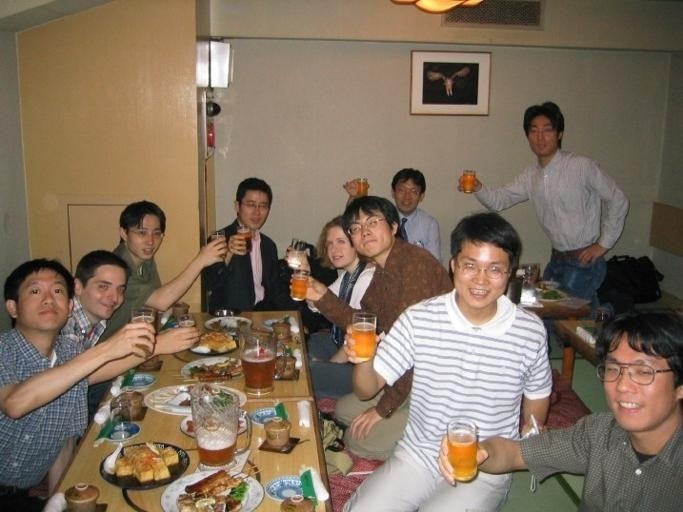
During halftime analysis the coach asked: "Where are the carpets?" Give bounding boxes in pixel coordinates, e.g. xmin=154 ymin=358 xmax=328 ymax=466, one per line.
xmin=315 ymin=367 xmax=593 ymax=508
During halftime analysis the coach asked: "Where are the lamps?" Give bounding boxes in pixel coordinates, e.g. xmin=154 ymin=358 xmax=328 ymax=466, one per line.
xmin=393 ymin=0 xmax=484 ymax=13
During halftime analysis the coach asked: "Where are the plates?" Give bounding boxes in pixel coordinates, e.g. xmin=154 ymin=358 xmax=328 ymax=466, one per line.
xmin=161 ymin=471 xmax=265 ymax=512
xmin=258 ymin=436 xmax=300 ymax=454
xmin=188 ymin=333 xmax=240 ymax=356
xmin=62 ymin=504 xmax=108 ymax=512
xmin=133 ymin=407 xmax=147 ymax=420
xmin=203 ymin=316 xmax=252 ymax=333
xmin=103 ymin=421 xmax=142 ymax=441
xmin=181 ymin=357 xmax=245 ymax=381
xmin=263 ymin=476 xmax=313 ymax=502
xmin=100 ymin=442 xmax=190 ymax=490
xmin=275 ymin=369 xmax=300 ymax=381
xmin=540 ymin=291 xmax=567 ymax=301
xmin=249 ymin=408 xmax=289 ymax=425
xmin=264 ymin=319 xmax=297 ymax=328
xmin=136 ymin=361 xmax=162 ymax=370
xmin=144 ymin=383 xmax=248 ymax=417
xmin=180 ymin=416 xmax=248 ymax=438
xmin=194 ymin=448 xmax=251 ymax=473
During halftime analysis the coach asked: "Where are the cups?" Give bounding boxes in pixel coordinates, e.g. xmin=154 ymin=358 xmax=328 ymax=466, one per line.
xmin=177 ymin=314 xmax=197 ymax=348
xmin=292 ymin=239 xmax=306 ymax=268
xmin=63 ymin=483 xmax=100 ymax=512
xmin=240 ymin=332 xmax=275 ymax=397
xmin=522 ymin=264 xmax=539 ymax=285
xmin=216 ymin=309 xmax=234 ymax=326
xmin=141 ymin=355 xmax=159 ymax=369
xmin=273 ymin=320 xmax=290 ymax=343
xmin=263 ymin=417 xmax=291 ymax=447
xmin=235 ymin=226 xmax=252 ymax=254
xmin=279 ymin=496 xmax=314 ymax=512
xmin=275 ymin=355 xmax=297 ymax=377
xmin=130 ymin=305 xmax=157 ymax=342
xmin=462 ymin=169 xmax=475 ymax=193
xmin=192 ymin=391 xmax=240 ymax=472
xmin=447 ymin=419 xmax=477 ymax=482
xmin=210 ymin=229 xmax=226 ymax=261
xmin=354 ymin=178 xmax=368 ymax=197
xmin=352 ymin=312 xmax=377 ymax=360
xmin=110 ymin=399 xmax=132 ymax=436
xmin=291 ymin=269 xmax=309 ymax=301
xmin=172 ymin=301 xmax=189 ymax=320
xmin=111 ymin=390 xmax=144 ymax=418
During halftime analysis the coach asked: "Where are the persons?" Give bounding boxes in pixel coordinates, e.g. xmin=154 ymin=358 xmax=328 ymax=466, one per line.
xmin=285 ymin=214 xmax=376 ymax=399
xmin=102 ymin=202 xmax=227 ymax=345
xmin=459 ymin=101 xmax=628 ymax=355
xmin=62 ymin=250 xmax=201 ymax=413
xmin=208 ymin=178 xmax=309 ymax=311
xmin=438 ymin=310 xmax=682 ymax=510
xmin=342 ymin=213 xmax=552 ymax=511
xmin=344 ymin=169 xmax=442 ymax=261
xmin=289 ymin=196 xmax=453 ymax=462
xmin=1 ymin=259 xmax=157 ymax=511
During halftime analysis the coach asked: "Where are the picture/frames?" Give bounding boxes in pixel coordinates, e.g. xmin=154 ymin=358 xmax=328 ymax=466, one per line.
xmin=409 ymin=49 xmax=491 ymax=116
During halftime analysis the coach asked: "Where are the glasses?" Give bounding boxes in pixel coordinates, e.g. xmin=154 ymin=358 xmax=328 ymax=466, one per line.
xmin=453 ymin=262 xmax=515 ymax=280
xmin=240 ymin=200 xmax=269 ymax=210
xmin=347 ymin=214 xmax=387 ymax=234
xmin=395 ymin=186 xmax=421 ymax=196
xmin=595 ymin=360 xmax=678 ymax=388
xmin=127 ymin=228 xmax=167 ymax=238
xmin=526 ymin=127 xmax=557 ymax=138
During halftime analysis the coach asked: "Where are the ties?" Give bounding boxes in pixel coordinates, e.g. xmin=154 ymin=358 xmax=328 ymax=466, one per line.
xmin=397 ymin=218 xmax=408 ymax=244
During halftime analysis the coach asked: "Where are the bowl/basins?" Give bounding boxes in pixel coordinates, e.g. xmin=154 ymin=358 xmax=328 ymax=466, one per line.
xmin=537 ymin=280 xmax=560 ymax=290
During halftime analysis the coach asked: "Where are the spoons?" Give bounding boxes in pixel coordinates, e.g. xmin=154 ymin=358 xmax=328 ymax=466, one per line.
xmin=120 ymin=373 xmax=156 ymax=388
xmin=103 ymin=443 xmax=123 ymax=475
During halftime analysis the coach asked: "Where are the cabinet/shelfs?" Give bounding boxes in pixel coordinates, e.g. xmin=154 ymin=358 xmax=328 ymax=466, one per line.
xmin=16 ymin=0 xmax=217 ymax=313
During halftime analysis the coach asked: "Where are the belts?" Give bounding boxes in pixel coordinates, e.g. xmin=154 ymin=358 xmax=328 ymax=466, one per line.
xmin=551 ymin=248 xmax=584 ymax=257
xmin=1 ymin=484 xmax=28 ymax=496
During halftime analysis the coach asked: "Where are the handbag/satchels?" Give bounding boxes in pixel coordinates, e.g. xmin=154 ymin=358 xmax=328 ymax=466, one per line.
xmin=599 ymin=255 xmax=665 ymax=304
